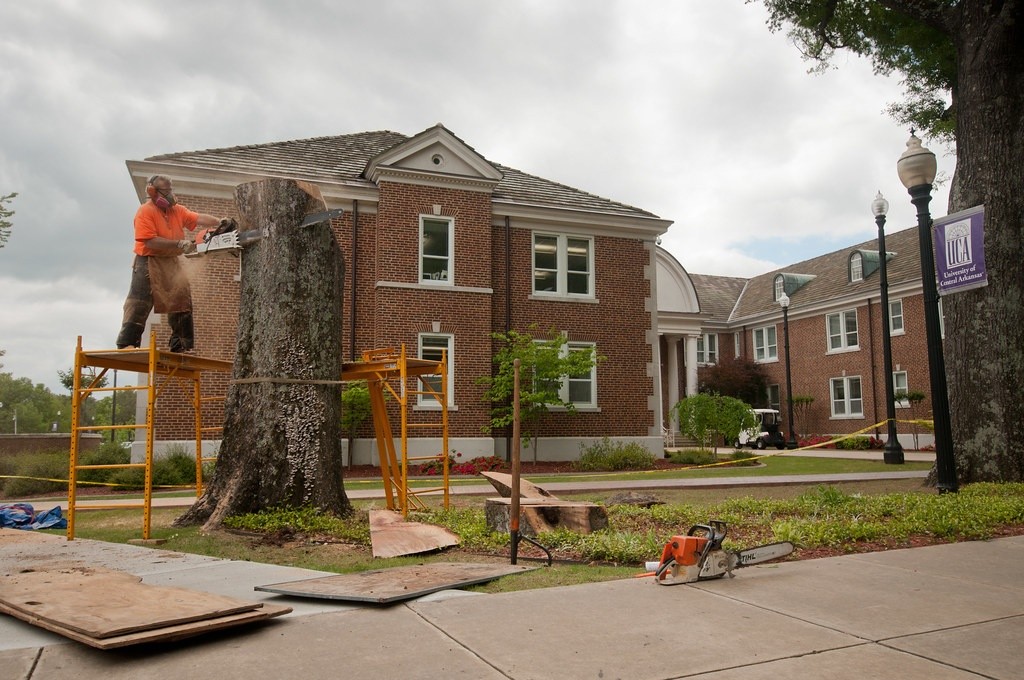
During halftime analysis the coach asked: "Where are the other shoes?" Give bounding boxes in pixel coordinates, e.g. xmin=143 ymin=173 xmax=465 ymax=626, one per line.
xmin=178 ymin=346 xmax=197 ymax=354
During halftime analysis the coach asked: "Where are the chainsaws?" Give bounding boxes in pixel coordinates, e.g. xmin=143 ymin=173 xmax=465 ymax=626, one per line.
xmin=183 ymin=208 xmax=344 ymax=260
xmin=654 ymin=519 xmax=795 ymax=586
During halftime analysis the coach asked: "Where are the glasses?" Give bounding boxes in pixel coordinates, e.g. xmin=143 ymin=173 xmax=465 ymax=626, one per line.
xmin=156 ymin=188 xmax=172 ymax=193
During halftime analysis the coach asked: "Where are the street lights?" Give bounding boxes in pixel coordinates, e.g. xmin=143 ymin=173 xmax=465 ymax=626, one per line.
xmin=897 ymin=126 xmax=962 ymax=493
xmin=778 ymin=292 xmax=799 ymax=449
xmin=870 ymin=189 xmax=907 ymax=465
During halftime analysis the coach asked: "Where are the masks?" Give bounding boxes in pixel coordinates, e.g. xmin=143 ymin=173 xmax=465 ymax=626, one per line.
xmin=155 ymin=193 xmax=177 ymax=210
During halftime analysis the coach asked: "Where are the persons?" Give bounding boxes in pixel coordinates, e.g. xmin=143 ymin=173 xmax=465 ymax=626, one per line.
xmin=115 ymin=175 xmax=239 ymax=355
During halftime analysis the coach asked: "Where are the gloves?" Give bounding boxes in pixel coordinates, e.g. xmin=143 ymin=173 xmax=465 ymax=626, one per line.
xmin=176 ymin=239 xmax=195 ymax=254
xmin=220 ymin=217 xmax=232 ymax=229
